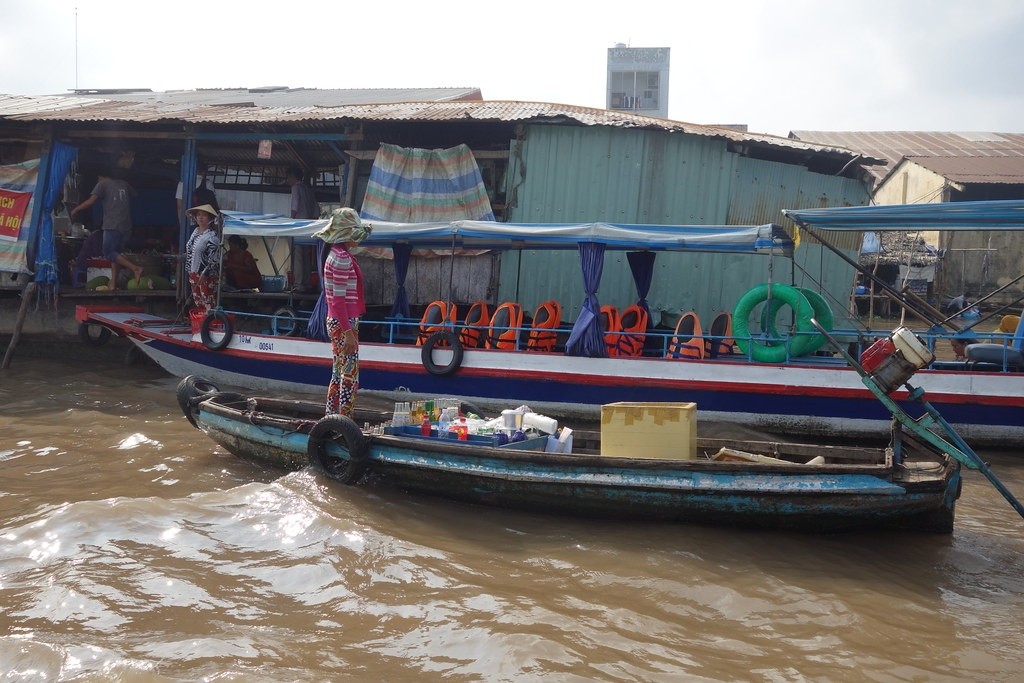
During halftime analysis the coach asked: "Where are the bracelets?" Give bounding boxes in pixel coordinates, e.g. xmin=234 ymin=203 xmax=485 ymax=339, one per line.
xmin=343 ymin=329 xmax=352 ymax=334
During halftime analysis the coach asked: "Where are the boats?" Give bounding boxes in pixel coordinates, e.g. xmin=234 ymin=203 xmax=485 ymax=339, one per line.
xmin=73 ymin=196 xmax=1024 ymax=454
xmin=176 ymin=319 xmax=1024 ymax=539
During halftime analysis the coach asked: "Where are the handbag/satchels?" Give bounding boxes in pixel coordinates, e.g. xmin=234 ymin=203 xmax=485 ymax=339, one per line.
xmin=195 ymin=172 xmax=220 ymax=214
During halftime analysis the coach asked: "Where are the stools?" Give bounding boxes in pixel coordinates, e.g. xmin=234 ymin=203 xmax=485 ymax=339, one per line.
xmin=72 ymin=269 xmax=87 ymax=288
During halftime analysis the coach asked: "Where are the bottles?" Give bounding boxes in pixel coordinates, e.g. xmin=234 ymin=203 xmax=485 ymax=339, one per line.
xmin=466 ymin=412 xmax=481 ymax=420
xmin=457 ymin=418 xmax=468 ymax=440
xmin=437 ymin=409 xmax=449 ymax=439
xmin=359 ymin=397 xmax=460 ymax=436
xmin=421 ymin=415 xmax=431 ymax=437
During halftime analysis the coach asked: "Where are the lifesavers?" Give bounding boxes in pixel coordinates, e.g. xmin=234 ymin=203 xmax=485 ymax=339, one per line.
xmin=175 ymin=374 xmax=220 ymax=430
xmin=421 ymin=331 xmax=463 ymax=377
xmin=270 ymin=306 xmax=300 ymax=337
xmin=307 ymin=414 xmax=366 ymax=485
xmin=201 ymin=311 xmax=233 ymax=351
xmin=760 ymin=288 xmax=834 ymax=357
xmin=732 ymin=284 xmax=814 ymax=362
xmin=79 ymin=319 xmax=110 ymax=346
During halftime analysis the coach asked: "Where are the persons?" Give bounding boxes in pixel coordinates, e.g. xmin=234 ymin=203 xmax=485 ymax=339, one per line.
xmin=170 ymin=161 xmax=216 ymax=289
xmin=312 ymin=208 xmax=372 ymax=432
xmin=947 ymin=292 xmax=972 ymax=318
xmin=853 ymin=280 xmax=876 ymax=315
xmin=185 ymin=204 xmax=220 ymax=311
xmin=71 ymin=168 xmax=143 ymax=290
xmin=286 ymin=167 xmax=323 ymax=292
xmin=222 ymin=235 xmax=262 ymax=292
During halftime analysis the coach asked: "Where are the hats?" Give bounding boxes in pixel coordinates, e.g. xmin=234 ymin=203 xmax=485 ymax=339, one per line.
xmin=188 ymin=204 xmax=218 ymax=216
xmin=311 ymin=207 xmax=372 ymax=244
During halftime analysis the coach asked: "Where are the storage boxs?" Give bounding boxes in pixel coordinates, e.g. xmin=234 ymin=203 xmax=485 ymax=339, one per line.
xmin=384 ymin=424 xmax=560 ymax=452
xmin=287 ymin=271 xmax=319 ymax=288
xmin=87 ymin=268 xmax=112 ymax=282
xmin=261 ymin=276 xmax=286 ymax=292
xmin=600 ymin=402 xmax=698 ymax=461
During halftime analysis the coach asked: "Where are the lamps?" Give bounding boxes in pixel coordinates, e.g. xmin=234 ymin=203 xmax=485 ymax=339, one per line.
xmin=258 ymin=140 xmax=272 ymax=159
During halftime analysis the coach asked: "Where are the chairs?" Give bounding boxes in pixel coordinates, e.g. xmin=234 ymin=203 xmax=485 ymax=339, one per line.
xmin=964 ymin=310 xmax=1024 ymax=372
xmin=417 ymin=300 xmax=735 ymax=360
